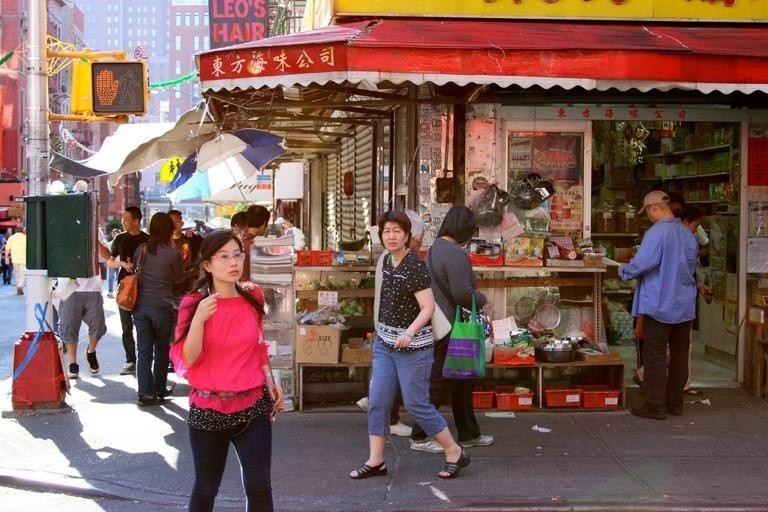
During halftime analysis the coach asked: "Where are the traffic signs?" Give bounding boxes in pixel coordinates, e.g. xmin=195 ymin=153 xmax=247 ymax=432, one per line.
xmin=87 ymin=60 xmax=149 ymax=118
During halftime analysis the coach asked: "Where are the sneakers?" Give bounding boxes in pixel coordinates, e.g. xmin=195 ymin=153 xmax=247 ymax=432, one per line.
xmin=67 ymin=346 xmax=99 ymax=378
xmin=355 ymin=397 xmax=370 ymax=413
xmin=458 ymin=435 xmax=495 ymax=447
xmin=119 ymin=362 xmax=136 ymax=374
xmin=389 ymin=421 xmax=445 ymax=453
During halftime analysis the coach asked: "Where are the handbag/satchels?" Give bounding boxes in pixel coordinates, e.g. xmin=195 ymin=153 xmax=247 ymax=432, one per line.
xmin=116 ymin=246 xmax=146 ymax=311
xmin=441 ymin=292 xmax=492 ymax=379
xmin=430 ymin=302 xmax=452 ymax=341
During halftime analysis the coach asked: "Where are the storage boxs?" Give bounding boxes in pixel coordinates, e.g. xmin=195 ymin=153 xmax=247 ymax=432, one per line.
xmin=504 ymin=256 xmax=544 ymax=268
xmin=614 ymin=244 xmax=633 ymax=263
xmin=541 ymin=379 xmax=581 ymax=407
xmin=493 ymin=381 xmax=534 ymax=410
xmin=469 ymin=382 xmax=495 ymax=409
xmin=581 ymin=380 xmax=622 ymax=407
xmin=469 ymin=252 xmax=503 ymax=267
xmin=546 ymin=258 xmax=584 ymax=267
xmin=341 ymin=345 xmax=374 ymax=365
xmin=494 ymin=343 xmax=537 ymax=366
xmin=296 ymin=322 xmax=340 ymax=366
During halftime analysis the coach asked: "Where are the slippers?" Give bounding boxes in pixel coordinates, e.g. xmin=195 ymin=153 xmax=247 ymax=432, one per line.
xmin=684 ymin=387 xmax=703 ymax=396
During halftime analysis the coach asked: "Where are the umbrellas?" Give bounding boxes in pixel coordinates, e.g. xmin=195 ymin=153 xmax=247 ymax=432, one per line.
xmin=167 ymin=129 xmax=288 ymax=207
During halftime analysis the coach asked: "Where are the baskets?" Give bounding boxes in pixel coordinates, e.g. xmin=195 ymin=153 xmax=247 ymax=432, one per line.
xmin=472 ymin=385 xmax=535 ymax=411
xmin=544 ymin=383 xmax=620 ymax=409
xmin=297 ymin=250 xmax=333 ymax=266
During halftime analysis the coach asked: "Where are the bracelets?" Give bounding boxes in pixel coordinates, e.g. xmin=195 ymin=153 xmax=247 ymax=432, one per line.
xmin=403 ymin=332 xmax=413 ymax=340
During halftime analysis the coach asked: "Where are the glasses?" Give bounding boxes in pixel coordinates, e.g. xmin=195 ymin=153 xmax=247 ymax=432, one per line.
xmin=213 ymin=252 xmax=245 ymax=262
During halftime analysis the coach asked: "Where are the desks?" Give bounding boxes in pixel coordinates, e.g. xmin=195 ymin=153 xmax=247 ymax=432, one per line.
xmin=746 ymin=319 xmax=768 ymax=399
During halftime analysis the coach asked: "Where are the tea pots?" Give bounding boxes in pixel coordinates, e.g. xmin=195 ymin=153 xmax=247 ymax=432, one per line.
xmin=514 ymin=173 xmax=557 ymax=210
xmin=486 ymin=185 xmax=510 ymax=205
xmin=474 ymin=191 xmax=504 ymax=227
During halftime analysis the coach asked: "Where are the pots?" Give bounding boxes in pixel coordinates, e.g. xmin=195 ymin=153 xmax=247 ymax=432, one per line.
xmin=536 ymin=335 xmax=578 ymax=362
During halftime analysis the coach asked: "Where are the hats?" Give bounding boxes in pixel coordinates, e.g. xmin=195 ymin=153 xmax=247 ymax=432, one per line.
xmin=637 ymin=190 xmax=671 ymax=215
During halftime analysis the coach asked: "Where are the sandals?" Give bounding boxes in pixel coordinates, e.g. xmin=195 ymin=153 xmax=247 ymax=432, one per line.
xmin=437 ymin=446 xmax=470 ymax=478
xmin=349 ymin=459 xmax=387 ymax=479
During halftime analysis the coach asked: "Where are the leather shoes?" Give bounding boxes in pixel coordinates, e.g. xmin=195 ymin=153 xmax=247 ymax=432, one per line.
xmin=632 ymin=404 xmax=683 ymax=419
xmin=137 ymin=385 xmax=173 ymax=407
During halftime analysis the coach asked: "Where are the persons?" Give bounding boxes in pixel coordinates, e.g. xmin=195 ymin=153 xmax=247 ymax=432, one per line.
xmin=0 ymin=204 xmax=272 ymax=406
xmin=633 ymin=204 xmax=714 ymax=395
xmin=174 ymin=159 xmax=180 ymax=171
xmin=618 ymin=188 xmax=698 ymax=420
xmin=168 ymin=227 xmax=284 ymax=511
xmin=355 ymin=210 xmax=425 ymax=437
xmin=169 ymin=161 xmax=175 ymax=176
xmin=347 ymin=210 xmax=473 ymax=480
xmin=408 ymin=205 xmax=495 ymax=454
xmin=636 ymin=192 xmax=711 ymax=369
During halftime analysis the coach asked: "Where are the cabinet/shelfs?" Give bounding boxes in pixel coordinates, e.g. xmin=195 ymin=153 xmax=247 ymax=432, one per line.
xmin=586 ymin=232 xmax=642 ymax=299
xmin=640 ymin=125 xmax=741 ymax=238
xmin=297 ymin=266 xmax=627 ymax=408
